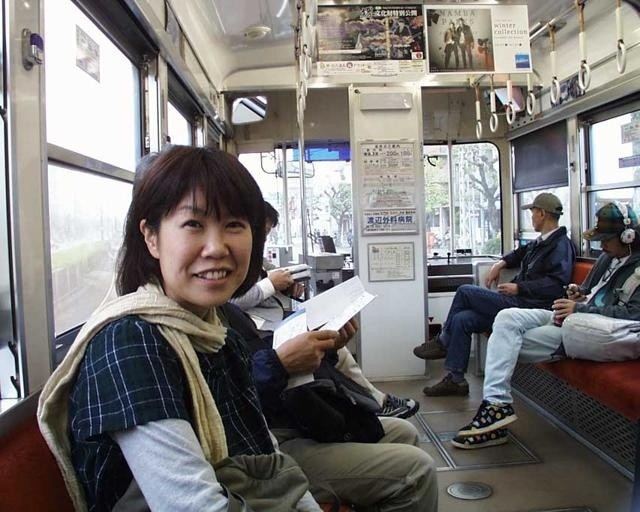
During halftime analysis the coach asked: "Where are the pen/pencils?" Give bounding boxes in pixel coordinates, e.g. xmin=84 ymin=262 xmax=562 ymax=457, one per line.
xmin=290 ymin=268 xmax=308 ymax=275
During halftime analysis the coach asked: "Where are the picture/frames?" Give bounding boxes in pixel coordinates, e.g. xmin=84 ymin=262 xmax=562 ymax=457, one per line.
xmin=367 ymin=242 xmax=415 ymax=283
xmin=357 ymin=140 xmax=419 ymax=237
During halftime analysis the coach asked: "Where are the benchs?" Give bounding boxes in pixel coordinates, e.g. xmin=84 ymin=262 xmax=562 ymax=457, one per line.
xmin=480 ymin=262 xmax=638 ymax=481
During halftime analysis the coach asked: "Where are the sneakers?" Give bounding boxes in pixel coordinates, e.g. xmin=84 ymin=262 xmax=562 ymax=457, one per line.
xmin=451 ymin=429 xmax=509 ymax=449
xmin=413 ymin=333 xmax=449 ymax=359
xmin=457 ymin=401 xmax=519 ymax=437
xmin=423 ymin=373 xmax=469 ymax=396
xmin=374 ymin=393 xmax=419 ymax=418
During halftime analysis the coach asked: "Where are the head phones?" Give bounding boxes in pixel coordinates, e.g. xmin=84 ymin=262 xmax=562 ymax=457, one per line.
xmin=611 ymin=200 xmax=635 ymax=244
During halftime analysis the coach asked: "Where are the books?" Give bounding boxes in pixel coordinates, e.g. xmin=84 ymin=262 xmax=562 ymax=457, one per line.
xmin=265 ymin=262 xmax=312 ymax=284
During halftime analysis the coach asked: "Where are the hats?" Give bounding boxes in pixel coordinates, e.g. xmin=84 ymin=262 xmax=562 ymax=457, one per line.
xmin=582 ymin=203 xmax=638 ymax=241
xmin=520 ymin=193 xmax=564 ymax=215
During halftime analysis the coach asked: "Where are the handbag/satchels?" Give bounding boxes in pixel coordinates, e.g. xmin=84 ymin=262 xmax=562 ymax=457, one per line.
xmin=110 ymin=452 xmax=308 ymax=511
xmin=285 ymin=367 xmax=385 ymax=443
xmin=562 ymin=312 xmax=640 ymax=362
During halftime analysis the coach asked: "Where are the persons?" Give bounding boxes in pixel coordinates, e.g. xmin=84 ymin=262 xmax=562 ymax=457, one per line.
xmin=449 ymin=198 xmax=639 ymax=451
xmin=34 ymin=143 xmax=331 ymax=512
xmin=133 ymin=151 xmax=440 ymax=511
xmin=225 ymin=200 xmax=424 ymax=419
xmin=412 ymin=193 xmax=575 ymax=402
xmin=454 ymin=17 xmax=476 ymax=70
xmin=443 ymin=20 xmax=461 ymax=70
